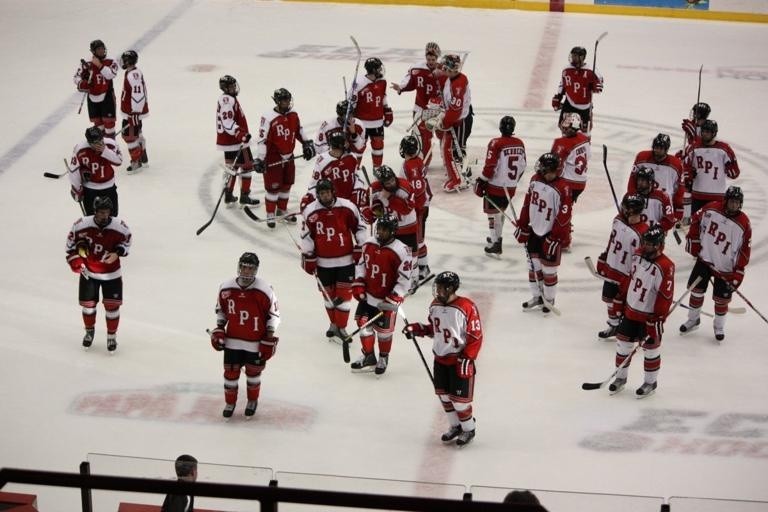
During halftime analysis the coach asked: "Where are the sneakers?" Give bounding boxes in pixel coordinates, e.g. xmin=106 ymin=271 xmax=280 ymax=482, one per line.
xmin=417 ymin=265 xmax=430 ymax=281
xmin=222 ymin=322 xmax=474 ymax=445
xmin=83 ymin=326 xmax=94 ymax=347
xmin=522 ymin=297 xmax=724 ymax=396
xmin=266 ymin=213 xmax=276 ymax=228
xmin=224 ymin=187 xmax=238 ymax=203
xmin=485 ymin=241 xmax=503 ymax=254
xmin=486 ymin=236 xmax=494 ymax=244
xmin=276 ymin=209 xmax=298 ymax=224
xmin=107 ymin=332 xmax=116 ymax=351
xmin=240 ymin=191 xmax=260 ymax=208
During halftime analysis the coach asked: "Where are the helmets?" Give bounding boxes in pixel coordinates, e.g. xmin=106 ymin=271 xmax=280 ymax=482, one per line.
xmin=567 ymin=46 xmax=588 ymax=68
xmin=399 ymin=135 xmax=422 ymax=159
xmin=651 ymin=133 xmax=672 ymax=158
xmin=639 ymin=224 xmax=665 ymax=260
xmin=377 ymin=215 xmax=398 ymax=245
xmin=85 ymin=39 xmax=138 ymax=213
xmin=498 ymin=116 xmax=514 ymax=136
xmin=425 ymin=45 xmax=441 ymax=58
xmin=364 ymin=57 xmax=386 ymax=78
xmin=219 ymin=75 xmax=240 ymax=97
xmin=440 ymin=54 xmax=462 ymax=73
xmin=327 ymin=130 xmax=346 ymax=149
xmin=621 ymin=192 xmax=645 ymax=218
xmin=336 ymin=99 xmax=353 ymax=124
xmin=691 ymin=104 xmax=711 ymax=122
xmin=238 ymin=251 xmax=260 ymax=286
xmin=559 ymin=113 xmax=582 ymax=137
xmin=316 ymin=178 xmax=337 ymax=207
xmin=634 ymin=166 xmax=656 ymax=195
xmin=272 ymin=87 xmax=293 ymax=112
xmin=432 ymin=271 xmax=460 ymax=302
xmin=538 ymin=153 xmax=561 ymax=181
xmin=725 ymin=186 xmax=743 ymax=214
xmin=375 ymin=165 xmax=399 ymax=193
xmin=700 ymin=120 xmax=719 ymax=144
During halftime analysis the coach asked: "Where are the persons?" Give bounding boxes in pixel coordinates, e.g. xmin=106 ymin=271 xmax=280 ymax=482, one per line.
xmin=627 ymin=133 xmax=685 ymax=230
xmin=349 ymin=57 xmax=393 ymax=174
xmin=503 ymin=490 xmax=540 ymax=506
xmin=551 ymin=47 xmax=603 ymax=145
xmin=312 ymin=100 xmax=433 ymax=293
xmin=402 ymin=272 xmax=483 ymax=446
xmin=550 ymin=112 xmax=589 ymax=249
xmin=301 ymin=179 xmax=367 ymax=343
xmin=679 ymin=186 xmax=752 ymax=345
xmin=65 ymin=196 xmax=132 ymax=352
xmin=513 ymin=153 xmax=572 ymax=313
xmin=252 ymin=88 xmax=316 ymax=228
xmin=216 ymin=75 xmax=260 ymax=206
xmin=211 ymin=252 xmax=280 ymax=418
xmin=160 ymin=455 xmax=198 ymax=511
xmin=677 ymin=103 xmax=740 ymax=214
xmin=597 ymin=191 xmax=649 ymax=341
xmin=74 ymin=39 xmax=118 ymax=140
xmin=474 ymin=115 xmax=527 ymax=255
xmin=70 ymin=126 xmax=123 ymax=217
xmin=119 ymin=50 xmax=148 ymax=172
xmin=634 ymin=166 xmax=674 ymax=252
xmin=390 ymin=44 xmax=474 ymax=190
xmin=351 ymin=215 xmax=413 ymax=375
xmin=607 ymin=225 xmax=675 ymax=395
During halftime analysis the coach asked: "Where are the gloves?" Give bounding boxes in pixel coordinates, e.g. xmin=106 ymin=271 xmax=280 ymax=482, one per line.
xmin=352 ymin=277 xmax=368 ymax=302
xmin=302 ymin=141 xmax=316 ymax=161
xmin=674 ymin=203 xmax=684 ymax=221
xmin=252 ymin=158 xmax=266 ymax=173
xmin=301 ymin=252 xmax=318 ymax=275
xmin=384 ymin=293 xmax=404 ymax=302
xmin=353 ymin=245 xmax=364 ymax=264
xmin=685 ymin=235 xmax=703 ymax=256
xmin=373 ymin=196 xmax=388 ymax=217
xmin=552 ymin=94 xmax=562 ymax=111
xmin=474 ymin=176 xmax=489 ymax=198
xmin=383 ymin=111 xmax=393 ymax=127
xmin=236 ymin=131 xmax=251 ymax=143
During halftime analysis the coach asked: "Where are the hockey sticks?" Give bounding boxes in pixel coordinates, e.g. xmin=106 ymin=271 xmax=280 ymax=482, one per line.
xmin=502 ymin=182 xmax=563 ymax=314
xmin=705 ymin=269 xmax=745 ymax=317
xmin=585 ymin=33 xmax=606 ymax=159
xmin=602 ymin=145 xmax=621 ymax=216
xmin=584 ymin=256 xmax=716 ymax=320
xmin=694 ymin=248 xmax=768 ymax=328
xmin=691 ymin=64 xmax=702 ymax=171
xmin=465 ymin=158 xmax=517 ymax=227
xmin=581 ymin=264 xmax=705 ymax=389
